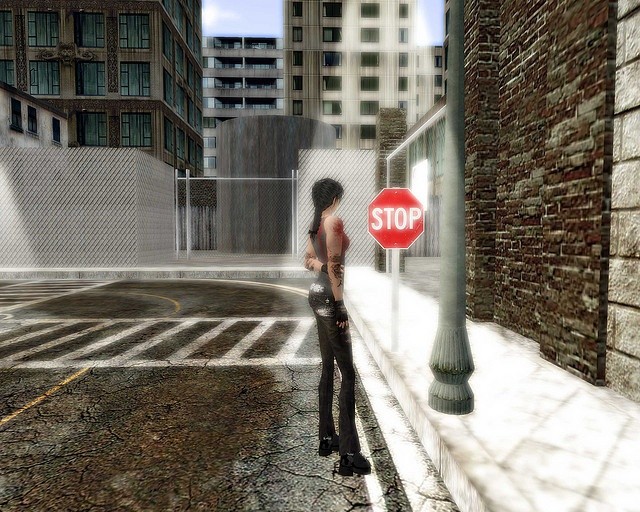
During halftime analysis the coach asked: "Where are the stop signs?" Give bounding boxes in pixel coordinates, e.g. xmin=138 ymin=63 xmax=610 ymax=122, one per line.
xmin=368 ymin=188 xmax=425 ymax=250
